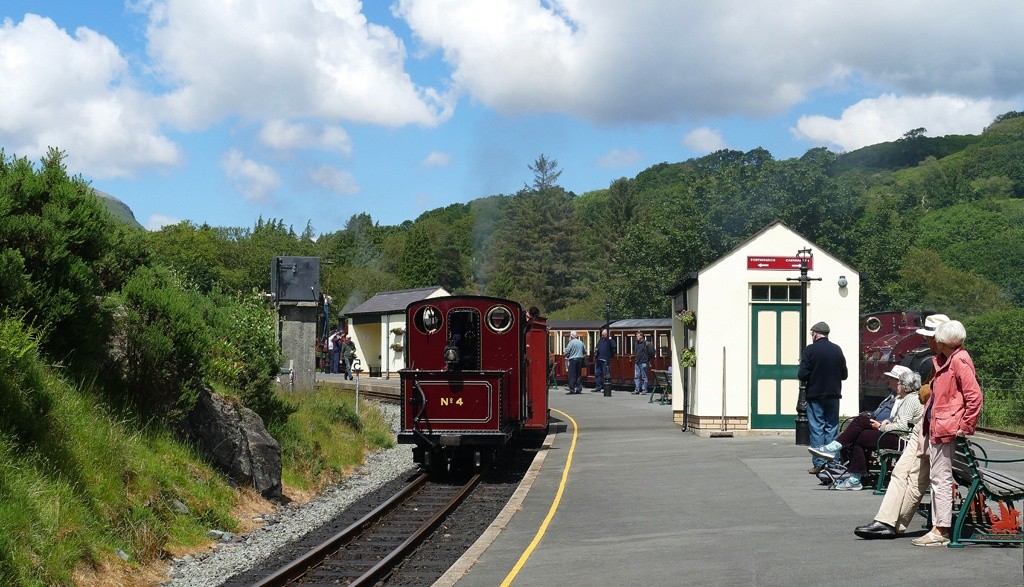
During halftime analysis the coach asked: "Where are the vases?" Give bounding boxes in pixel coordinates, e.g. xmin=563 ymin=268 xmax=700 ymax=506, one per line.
xmin=687 ymin=319 xmax=697 ymax=331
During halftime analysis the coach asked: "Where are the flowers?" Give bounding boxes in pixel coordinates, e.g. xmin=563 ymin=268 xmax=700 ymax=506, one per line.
xmin=675 ymin=309 xmax=696 ymax=326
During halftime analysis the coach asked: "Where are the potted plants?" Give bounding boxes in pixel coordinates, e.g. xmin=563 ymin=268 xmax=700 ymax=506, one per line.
xmin=389 ymin=343 xmax=404 ymax=352
xmin=390 ymin=328 xmax=406 ymax=335
xmin=679 ymin=345 xmax=696 ymax=368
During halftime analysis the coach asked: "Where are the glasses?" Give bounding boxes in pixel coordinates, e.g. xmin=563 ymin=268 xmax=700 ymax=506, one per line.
xmin=810 ymin=332 xmax=813 ymax=335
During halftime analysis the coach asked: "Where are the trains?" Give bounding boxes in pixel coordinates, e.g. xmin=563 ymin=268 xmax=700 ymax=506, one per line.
xmin=316 ymin=316 xmax=348 ymax=371
xmin=860 ymin=309 xmax=936 ymax=397
xmin=550 ymin=320 xmax=672 ymax=388
xmin=397 ymin=295 xmax=550 ymax=473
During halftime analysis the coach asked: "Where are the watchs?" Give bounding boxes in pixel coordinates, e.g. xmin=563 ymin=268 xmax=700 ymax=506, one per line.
xmin=878 ymin=425 xmax=881 ymax=430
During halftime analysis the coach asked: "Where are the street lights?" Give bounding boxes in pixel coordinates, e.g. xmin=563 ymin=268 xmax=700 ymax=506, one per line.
xmin=604 ymin=300 xmax=612 ymax=397
xmin=324 ymin=260 xmax=334 ymax=373
xmin=787 ymin=245 xmax=822 ymax=445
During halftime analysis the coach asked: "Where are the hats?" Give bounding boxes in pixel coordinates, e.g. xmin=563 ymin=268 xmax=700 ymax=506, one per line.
xmin=916 ymin=314 xmax=950 ymax=337
xmin=810 ymin=322 xmax=830 ymax=335
xmin=884 ymin=365 xmax=912 ymax=380
xmin=346 ymin=334 xmax=351 ymax=338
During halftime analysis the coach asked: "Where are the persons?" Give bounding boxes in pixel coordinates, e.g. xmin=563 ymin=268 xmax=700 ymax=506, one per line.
xmin=565 ymin=331 xmax=586 ymax=395
xmin=591 ymin=329 xmax=616 ymax=392
xmin=630 ymin=332 xmax=652 ymax=395
xmin=331 ymin=333 xmax=356 ymax=381
xmin=798 ymin=322 xmax=848 ymax=474
xmin=808 ymin=313 xmax=984 ymax=547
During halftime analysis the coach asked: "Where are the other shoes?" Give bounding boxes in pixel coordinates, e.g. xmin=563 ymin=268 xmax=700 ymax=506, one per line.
xmin=835 ymin=477 xmax=863 ymax=491
xmin=911 ymin=532 xmax=951 ymax=547
xmin=809 ymin=467 xmax=820 ymax=474
xmin=349 ymin=376 xmax=353 ymax=380
xmin=808 ymin=446 xmax=836 ymax=459
xmin=631 ymin=390 xmax=639 ymax=394
xmin=591 ymin=388 xmax=601 ymax=392
xmin=566 ymin=391 xmax=581 ymax=395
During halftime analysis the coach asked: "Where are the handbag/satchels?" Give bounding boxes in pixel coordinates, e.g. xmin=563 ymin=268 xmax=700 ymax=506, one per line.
xmin=353 ymin=354 xmax=357 ymax=359
xmin=582 ymin=352 xmax=589 ymax=367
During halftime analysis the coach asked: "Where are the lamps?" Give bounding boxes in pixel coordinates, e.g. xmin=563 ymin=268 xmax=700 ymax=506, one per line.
xmin=838 ymin=276 xmax=848 ymax=288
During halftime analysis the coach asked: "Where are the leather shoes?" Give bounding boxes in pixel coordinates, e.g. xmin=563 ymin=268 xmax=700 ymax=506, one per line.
xmin=854 ymin=520 xmax=896 ymax=538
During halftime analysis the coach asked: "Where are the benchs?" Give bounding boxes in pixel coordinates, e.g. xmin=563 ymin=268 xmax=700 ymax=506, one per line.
xmin=947 ymin=436 xmax=1024 ymax=549
xmin=548 ymin=362 xmax=557 ymax=389
xmin=648 ymin=369 xmax=672 ymax=405
xmin=839 ymin=416 xmax=912 ymax=495
